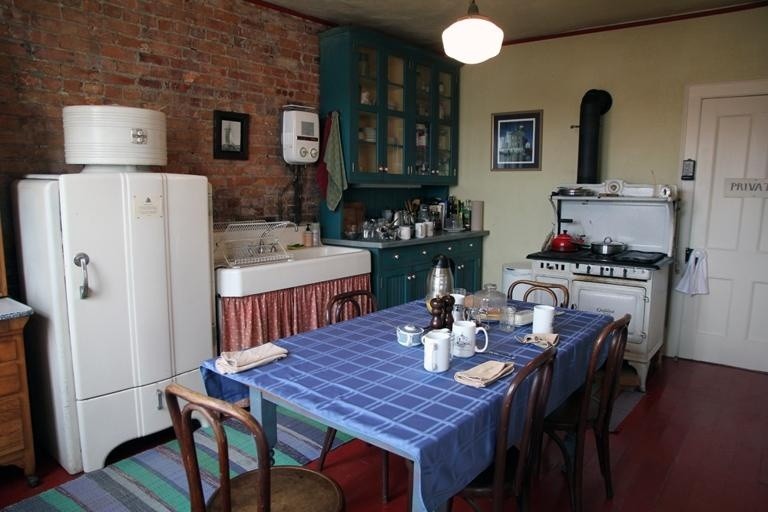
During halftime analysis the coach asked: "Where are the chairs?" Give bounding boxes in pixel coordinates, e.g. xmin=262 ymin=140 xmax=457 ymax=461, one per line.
xmin=325 ymin=290 xmax=375 ymax=327
xmin=164 ymin=383 xmax=343 ymax=512
xmin=405 ymin=351 xmax=556 ymax=511
xmin=519 ymin=314 xmax=632 ymax=510
xmin=507 ymin=281 xmax=570 ymax=308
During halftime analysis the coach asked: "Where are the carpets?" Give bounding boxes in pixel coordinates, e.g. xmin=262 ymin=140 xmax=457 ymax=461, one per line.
xmin=1 ymin=402 xmax=355 ymax=511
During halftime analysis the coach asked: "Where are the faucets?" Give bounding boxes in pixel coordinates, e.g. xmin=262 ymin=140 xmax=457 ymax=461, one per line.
xmin=257 ymin=221 xmax=298 ymax=254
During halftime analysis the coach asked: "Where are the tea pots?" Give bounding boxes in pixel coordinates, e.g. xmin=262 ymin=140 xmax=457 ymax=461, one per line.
xmin=549 ymin=229 xmax=584 ymax=252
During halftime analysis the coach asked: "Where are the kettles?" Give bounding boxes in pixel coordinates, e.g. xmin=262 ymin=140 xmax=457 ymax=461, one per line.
xmin=426 ymin=253 xmax=455 ymax=298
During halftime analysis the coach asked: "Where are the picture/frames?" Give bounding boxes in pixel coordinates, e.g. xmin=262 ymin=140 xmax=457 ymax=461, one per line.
xmin=213 ymin=110 xmax=249 ymax=159
xmin=489 ymin=109 xmax=543 ymax=172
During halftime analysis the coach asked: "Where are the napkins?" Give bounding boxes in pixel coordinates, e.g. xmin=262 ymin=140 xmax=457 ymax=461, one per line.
xmin=453 ymin=358 xmax=515 ymax=388
xmin=215 ymin=341 xmax=289 ymax=374
xmin=514 ymin=330 xmax=560 ymax=347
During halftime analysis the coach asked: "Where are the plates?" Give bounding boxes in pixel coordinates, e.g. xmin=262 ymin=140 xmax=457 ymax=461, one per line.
xmin=359 ymin=127 xmax=377 ymax=143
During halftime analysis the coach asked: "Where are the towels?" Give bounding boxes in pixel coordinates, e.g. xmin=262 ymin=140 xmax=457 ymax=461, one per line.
xmin=674 ymin=248 xmax=710 ymax=295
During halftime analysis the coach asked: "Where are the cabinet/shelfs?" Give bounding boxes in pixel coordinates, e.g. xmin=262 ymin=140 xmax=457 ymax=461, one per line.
xmin=433 ymin=238 xmax=474 ymax=294
xmin=384 ymin=241 xmax=433 ymax=306
xmin=0 ymin=296 xmax=33 ymax=487
xmin=356 ymin=44 xmax=405 ymax=177
xmin=414 ymin=60 xmax=457 ymax=184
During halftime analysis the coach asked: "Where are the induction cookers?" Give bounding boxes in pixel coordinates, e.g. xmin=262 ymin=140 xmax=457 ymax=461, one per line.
xmin=527 ymin=249 xmax=662 ymax=265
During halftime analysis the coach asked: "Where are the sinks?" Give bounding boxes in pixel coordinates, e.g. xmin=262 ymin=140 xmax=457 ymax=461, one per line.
xmin=215 ymin=257 xmax=294 ymax=297
xmin=287 ymin=245 xmax=373 ymax=288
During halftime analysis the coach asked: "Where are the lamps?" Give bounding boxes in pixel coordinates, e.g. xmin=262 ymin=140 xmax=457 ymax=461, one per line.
xmin=441 ymin=0 xmax=504 ymax=66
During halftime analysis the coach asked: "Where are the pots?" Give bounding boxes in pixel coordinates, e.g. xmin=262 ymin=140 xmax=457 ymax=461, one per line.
xmin=590 ymin=237 xmax=627 ymax=256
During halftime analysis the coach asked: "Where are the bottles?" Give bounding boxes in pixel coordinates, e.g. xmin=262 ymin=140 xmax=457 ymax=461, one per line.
xmin=471 ymin=281 xmax=516 ymax=334
xmin=448 ymin=195 xmax=471 ymax=233
xmin=420 ymin=206 xmax=440 ymax=231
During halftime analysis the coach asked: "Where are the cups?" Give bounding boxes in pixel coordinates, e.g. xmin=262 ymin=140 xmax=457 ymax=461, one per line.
xmin=396 ymin=324 xmax=423 ymax=348
xmin=531 ymin=305 xmax=555 ymax=335
xmin=423 ymin=320 xmax=488 ymax=373
xmin=450 ymin=293 xmax=466 ymax=321
xmin=398 ymin=222 xmax=435 ymax=240
